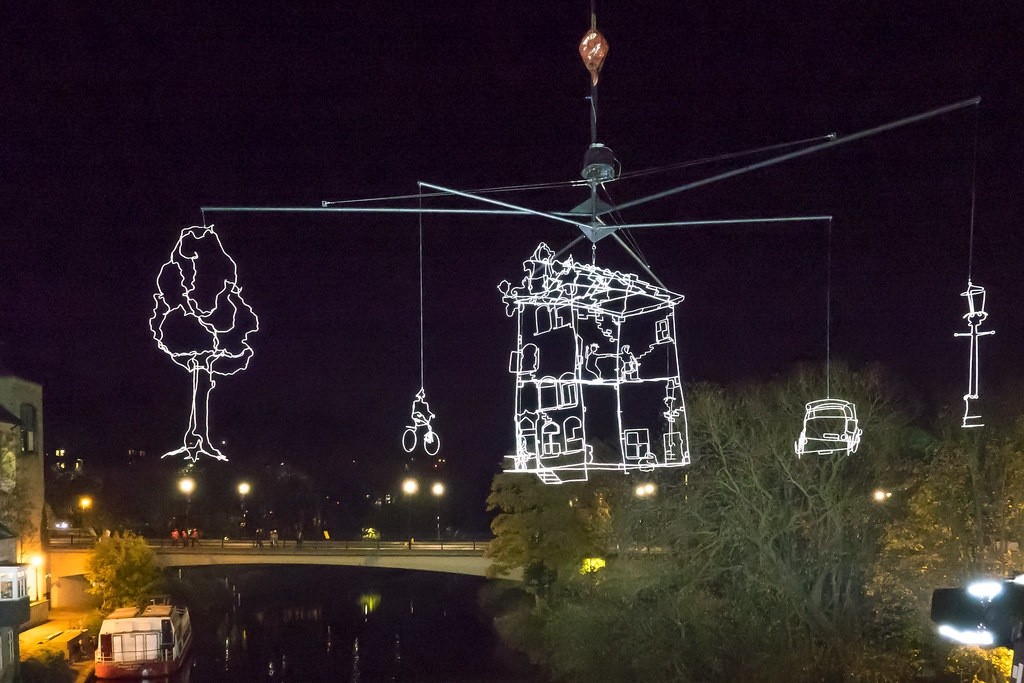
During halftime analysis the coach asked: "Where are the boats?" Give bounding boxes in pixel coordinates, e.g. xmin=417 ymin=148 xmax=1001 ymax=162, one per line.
xmin=94 ymin=596 xmax=196 ymax=679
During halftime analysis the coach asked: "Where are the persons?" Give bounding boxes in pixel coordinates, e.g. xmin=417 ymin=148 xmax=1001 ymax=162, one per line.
xmin=171 ymin=528 xmax=202 ymax=548
xmin=255 ymin=529 xmax=304 ymax=547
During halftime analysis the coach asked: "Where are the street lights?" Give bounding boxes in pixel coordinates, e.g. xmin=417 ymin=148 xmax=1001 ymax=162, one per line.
xmin=433 ymin=480 xmax=449 ymax=549
xmin=238 ymin=484 xmax=253 ymax=541
xmin=178 ymin=478 xmax=198 ymax=544
xmin=403 ymin=479 xmax=418 ymax=551
xmin=80 ymin=494 xmax=93 ymax=537
